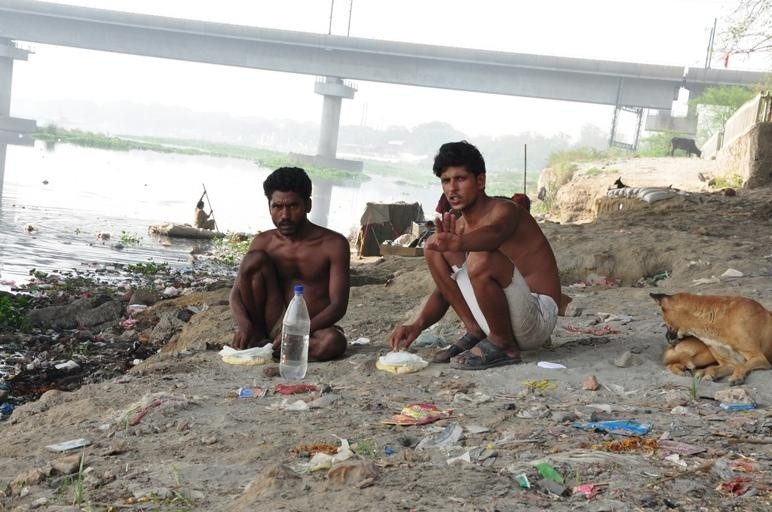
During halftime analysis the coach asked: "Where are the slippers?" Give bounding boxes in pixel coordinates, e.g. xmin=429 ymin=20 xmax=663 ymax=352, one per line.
xmin=449 ymin=339 xmax=523 ymax=368
xmin=431 ymin=333 xmax=478 ymax=362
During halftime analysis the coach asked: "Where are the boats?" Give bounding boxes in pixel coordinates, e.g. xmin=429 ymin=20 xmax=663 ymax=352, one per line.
xmin=147 ymin=222 xmax=227 ymax=239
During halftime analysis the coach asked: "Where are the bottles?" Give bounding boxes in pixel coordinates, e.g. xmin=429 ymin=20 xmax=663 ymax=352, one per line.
xmin=279 ymin=285 xmax=310 ymax=382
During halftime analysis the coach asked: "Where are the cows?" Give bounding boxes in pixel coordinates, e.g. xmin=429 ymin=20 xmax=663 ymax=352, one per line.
xmin=669 ymin=137 xmax=702 ymax=158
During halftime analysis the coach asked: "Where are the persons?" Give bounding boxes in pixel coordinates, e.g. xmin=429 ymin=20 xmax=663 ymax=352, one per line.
xmin=194 ymin=190 xmax=215 ymax=231
xmin=229 ymin=167 xmax=350 ymax=363
xmin=389 ymin=142 xmax=561 ymax=370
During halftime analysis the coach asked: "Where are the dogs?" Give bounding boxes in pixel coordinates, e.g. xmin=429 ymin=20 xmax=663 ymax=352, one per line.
xmin=647 ymin=291 xmax=772 ymax=387
